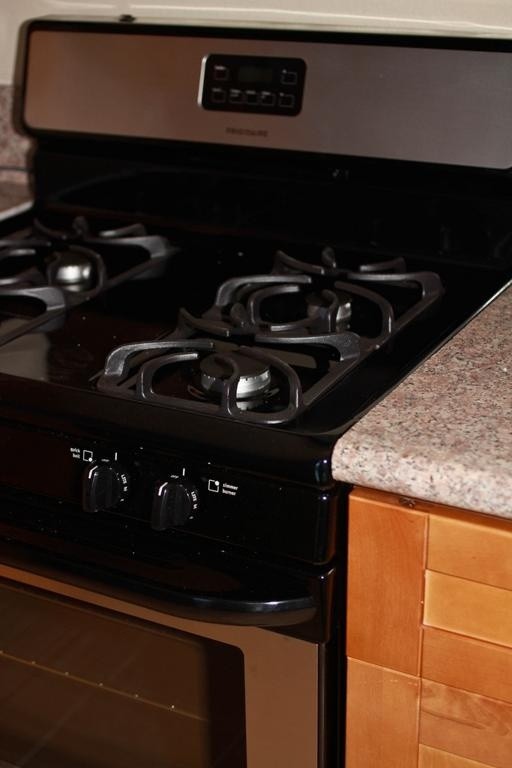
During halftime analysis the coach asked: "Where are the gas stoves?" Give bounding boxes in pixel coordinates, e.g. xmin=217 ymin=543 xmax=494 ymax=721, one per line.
xmin=0 ymin=208 xmax=449 ymax=425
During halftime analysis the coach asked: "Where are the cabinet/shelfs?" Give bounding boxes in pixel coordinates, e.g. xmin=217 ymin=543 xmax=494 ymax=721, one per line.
xmin=344 ymin=487 xmax=512 ymax=768
